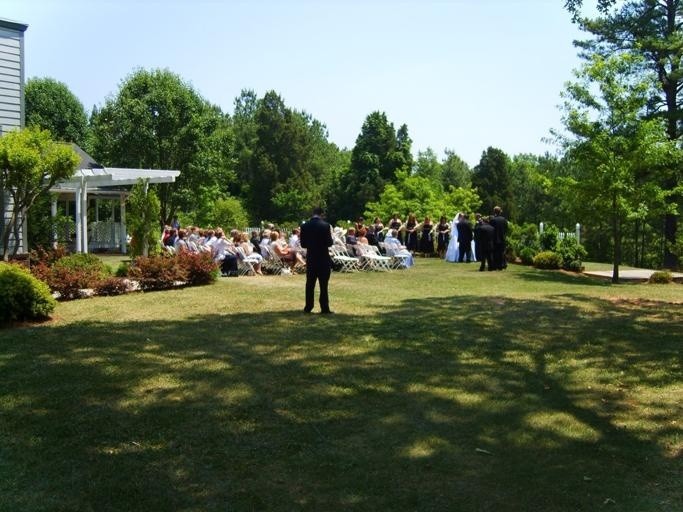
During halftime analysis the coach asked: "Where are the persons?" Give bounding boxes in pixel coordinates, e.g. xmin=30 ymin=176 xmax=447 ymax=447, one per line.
xmin=405 ymin=213 xmax=451 ymax=258
xmin=329 ymin=217 xmax=413 ymax=266
xmin=388 ymin=214 xmax=403 ymax=245
xmin=474 ymin=207 xmax=506 ymax=271
xmin=171 ymin=215 xmax=180 ymax=230
xmin=447 ymin=213 xmax=474 ymax=262
xmin=300 ymin=208 xmax=334 ymax=315
xmin=160 ymin=224 xmax=306 ymax=274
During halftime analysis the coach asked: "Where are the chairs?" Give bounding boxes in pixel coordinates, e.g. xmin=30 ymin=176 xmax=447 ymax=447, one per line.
xmin=159 ymin=238 xmax=409 ymax=277
